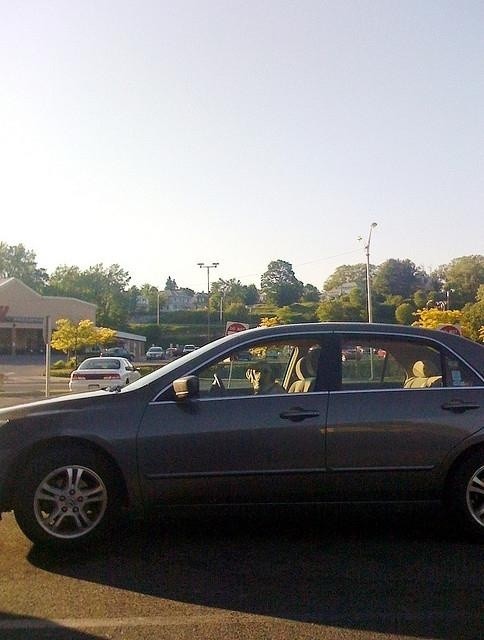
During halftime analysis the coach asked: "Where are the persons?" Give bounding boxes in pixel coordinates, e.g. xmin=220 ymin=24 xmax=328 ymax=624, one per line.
xmin=210 ymin=360 xmax=290 ymax=397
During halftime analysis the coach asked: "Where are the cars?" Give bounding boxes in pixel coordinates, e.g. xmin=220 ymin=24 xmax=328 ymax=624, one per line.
xmin=182 ymin=344 xmax=195 ymax=354
xmin=266 ymin=348 xmax=279 ymax=360
xmin=165 ymin=347 xmax=178 ymax=355
xmin=69 ymin=356 xmax=142 ymax=393
xmin=0 ymin=322 xmax=484 ymax=555
xmin=146 ymin=347 xmax=166 ymax=360
xmin=100 ymin=347 xmax=135 ymax=361
xmin=239 ymin=349 xmax=252 ymax=361
xmin=341 ymin=348 xmax=362 ymax=362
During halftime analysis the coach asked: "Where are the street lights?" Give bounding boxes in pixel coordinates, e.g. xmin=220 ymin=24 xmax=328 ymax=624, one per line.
xmin=156 ymin=293 xmax=164 ymax=325
xmin=356 ymin=222 xmax=378 ymax=380
xmin=197 ymin=262 xmax=220 ymax=341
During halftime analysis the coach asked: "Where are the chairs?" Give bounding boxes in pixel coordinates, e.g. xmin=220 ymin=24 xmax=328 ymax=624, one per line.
xmin=302 ymin=349 xmax=321 ymax=393
xmin=403 ymin=360 xmax=428 ymax=388
xmin=287 ymin=357 xmax=307 ymax=393
